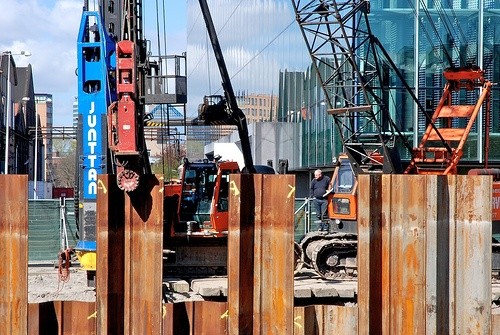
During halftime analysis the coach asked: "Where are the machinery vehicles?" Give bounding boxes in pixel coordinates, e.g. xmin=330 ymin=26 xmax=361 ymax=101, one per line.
xmin=56 ymin=1 xmax=306 ymax=284
xmin=280 ymin=0 xmax=500 ymax=283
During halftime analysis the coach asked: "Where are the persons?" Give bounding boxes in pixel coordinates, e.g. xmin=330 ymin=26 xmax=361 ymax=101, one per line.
xmin=310 ymin=169 xmax=331 ymax=234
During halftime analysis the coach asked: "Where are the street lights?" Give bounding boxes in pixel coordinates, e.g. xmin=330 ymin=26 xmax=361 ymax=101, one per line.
xmin=22 ymin=96 xmax=52 ymax=199
xmin=0 ymin=51 xmax=32 ymax=174
